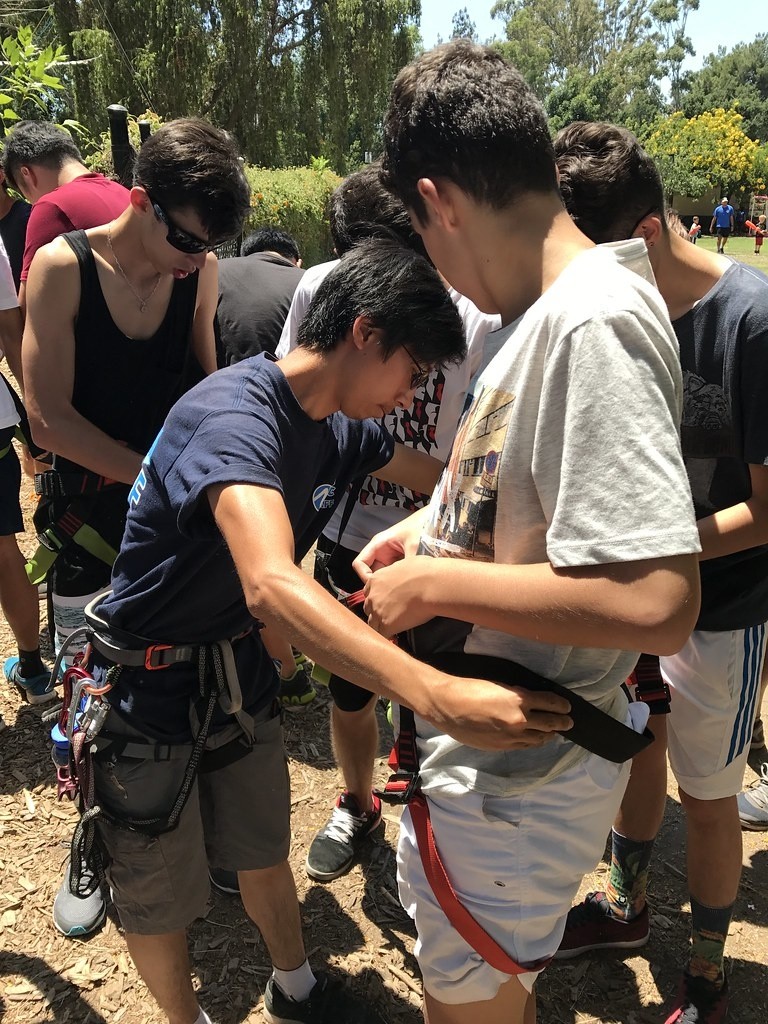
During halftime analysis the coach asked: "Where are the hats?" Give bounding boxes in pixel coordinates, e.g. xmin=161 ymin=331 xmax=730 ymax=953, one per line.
xmin=721 ymin=197 xmax=729 ymax=203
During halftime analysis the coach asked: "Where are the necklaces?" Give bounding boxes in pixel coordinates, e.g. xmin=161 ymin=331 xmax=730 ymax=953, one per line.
xmin=107 ymin=218 xmax=164 ymax=313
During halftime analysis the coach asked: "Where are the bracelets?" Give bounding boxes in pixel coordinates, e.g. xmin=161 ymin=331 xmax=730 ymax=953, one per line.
xmin=731 ymin=225 xmax=734 ymax=227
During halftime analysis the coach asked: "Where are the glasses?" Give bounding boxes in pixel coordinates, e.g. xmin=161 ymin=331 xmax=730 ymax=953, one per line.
xmin=143 ymin=185 xmax=230 ymax=255
xmin=392 ymin=338 xmax=431 ymax=390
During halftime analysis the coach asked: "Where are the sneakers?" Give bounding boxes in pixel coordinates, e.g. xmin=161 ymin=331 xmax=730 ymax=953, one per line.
xmin=658 ymin=972 xmax=729 ymax=1024
xmin=275 ymin=664 xmax=316 ymax=705
xmin=292 ymin=646 xmax=307 ymax=663
xmin=262 ymin=969 xmax=383 ymax=1024
xmin=208 ymin=860 xmax=239 ymax=893
xmin=551 ymin=890 xmax=650 ymax=959
xmin=734 ymin=762 xmax=768 ymax=832
xmin=52 ymin=850 xmax=107 ymax=936
xmin=3 ymin=657 xmax=57 ymax=703
xmin=307 ymin=786 xmax=383 ymax=880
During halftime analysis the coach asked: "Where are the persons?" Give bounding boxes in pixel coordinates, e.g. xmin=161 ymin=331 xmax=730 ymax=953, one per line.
xmin=53 ymin=236 xmax=575 ymax=1021
xmin=305 ymin=165 xmax=504 ymax=918
xmin=734 ymin=643 xmax=768 ymax=833
xmin=348 ymin=44 xmax=704 ymax=1021
xmin=688 ymin=216 xmax=701 ymax=245
xmin=0 ymin=115 xmax=305 ymax=939
xmin=754 ymin=215 xmax=768 ymax=256
xmin=548 ymin=121 xmax=768 ymax=1024
xmin=709 ymin=197 xmax=734 ymax=254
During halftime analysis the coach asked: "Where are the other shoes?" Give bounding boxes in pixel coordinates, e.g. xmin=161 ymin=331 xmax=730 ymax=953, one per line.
xmin=718 ymin=248 xmax=723 ymax=254
xmin=754 ymin=249 xmax=760 ymax=255
